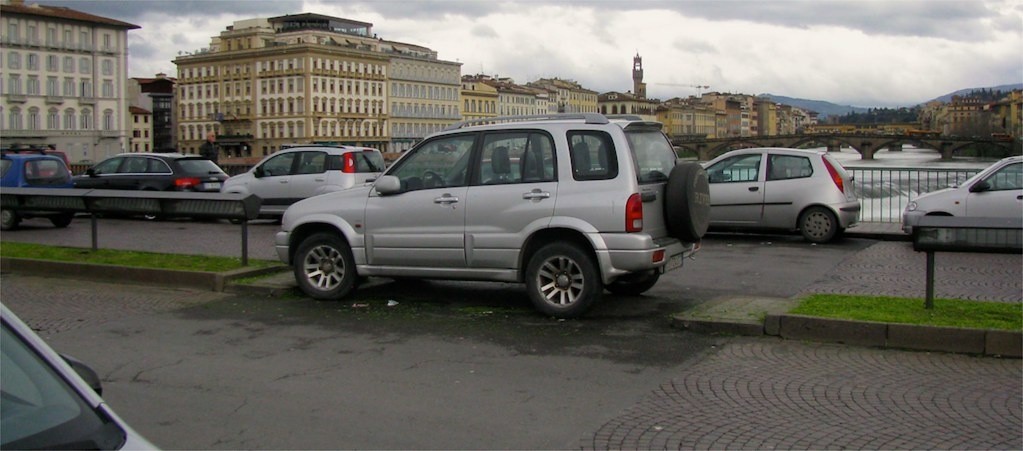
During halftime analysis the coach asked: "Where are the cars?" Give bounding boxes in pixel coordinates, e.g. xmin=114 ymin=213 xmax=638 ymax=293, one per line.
xmin=71 ymin=152 xmax=231 ymax=223
xmin=900 ymin=156 xmax=1023 ymax=251
xmin=700 ymin=148 xmax=860 ymax=243
xmin=0 ymin=303 xmax=163 ymax=451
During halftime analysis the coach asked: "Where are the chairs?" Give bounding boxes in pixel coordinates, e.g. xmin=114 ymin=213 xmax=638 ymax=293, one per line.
xmin=128 ymin=160 xmax=143 ymax=173
xmin=302 ymin=165 xmax=317 ymax=174
xmin=573 ymin=142 xmax=591 ymax=176
xmin=597 ymin=143 xmax=608 ymax=175
xmin=484 ymin=146 xmax=514 ymax=184
xmin=515 ymin=153 xmax=539 ymax=181
xmin=753 ymin=160 xmax=760 ymax=181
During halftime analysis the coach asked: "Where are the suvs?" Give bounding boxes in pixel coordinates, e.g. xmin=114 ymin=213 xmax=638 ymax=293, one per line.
xmin=220 ymin=143 xmax=387 ymax=225
xmin=0 ymin=144 xmax=77 ymax=232
xmin=274 ymin=112 xmax=711 ymax=319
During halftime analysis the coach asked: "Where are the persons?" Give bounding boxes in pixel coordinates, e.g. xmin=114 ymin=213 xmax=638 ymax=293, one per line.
xmin=199 ymin=130 xmax=219 ymax=166
